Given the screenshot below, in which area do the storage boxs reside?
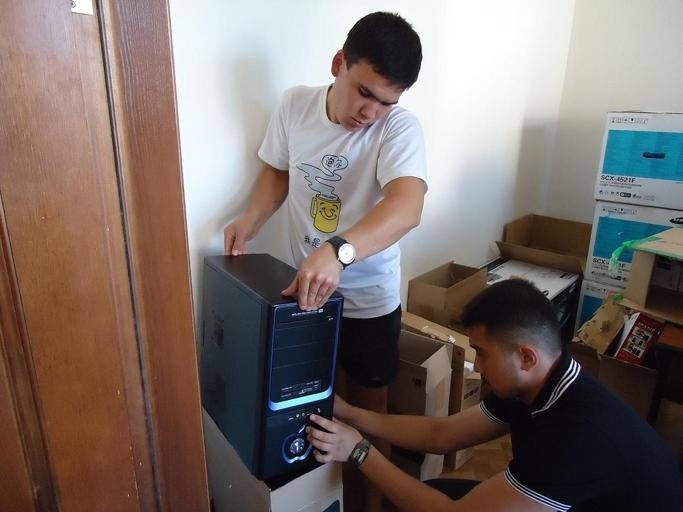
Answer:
[593,108,683,210]
[398,311,482,471]
[570,279,621,339]
[498,212,592,271]
[390,329,453,482]
[568,290,683,421]
[407,261,487,328]
[476,257,580,344]
[202,408,343,512]
[613,227,683,323]
[584,201,683,289]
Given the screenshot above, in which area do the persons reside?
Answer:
[222,10,427,512]
[304,276,683,511]
